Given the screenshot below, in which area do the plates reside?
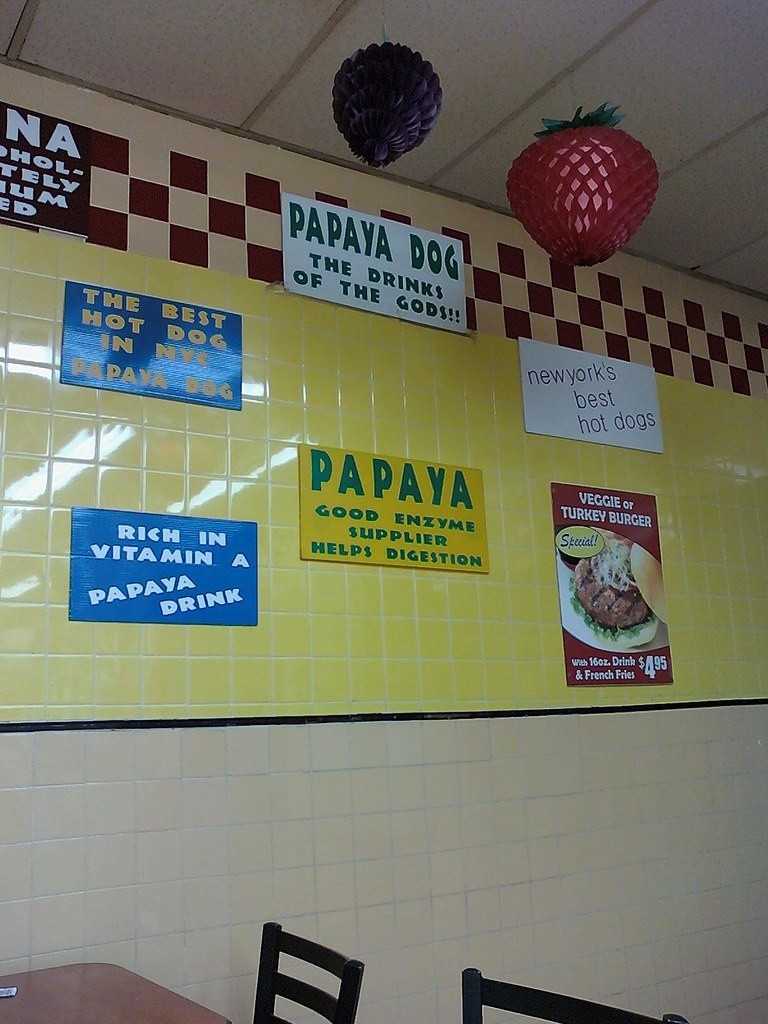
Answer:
[556,527,670,653]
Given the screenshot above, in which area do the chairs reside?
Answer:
[462,967,687,1024]
[249,922,366,1024]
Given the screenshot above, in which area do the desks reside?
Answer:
[0,962,232,1024]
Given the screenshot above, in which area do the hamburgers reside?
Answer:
[569,540,668,652]
